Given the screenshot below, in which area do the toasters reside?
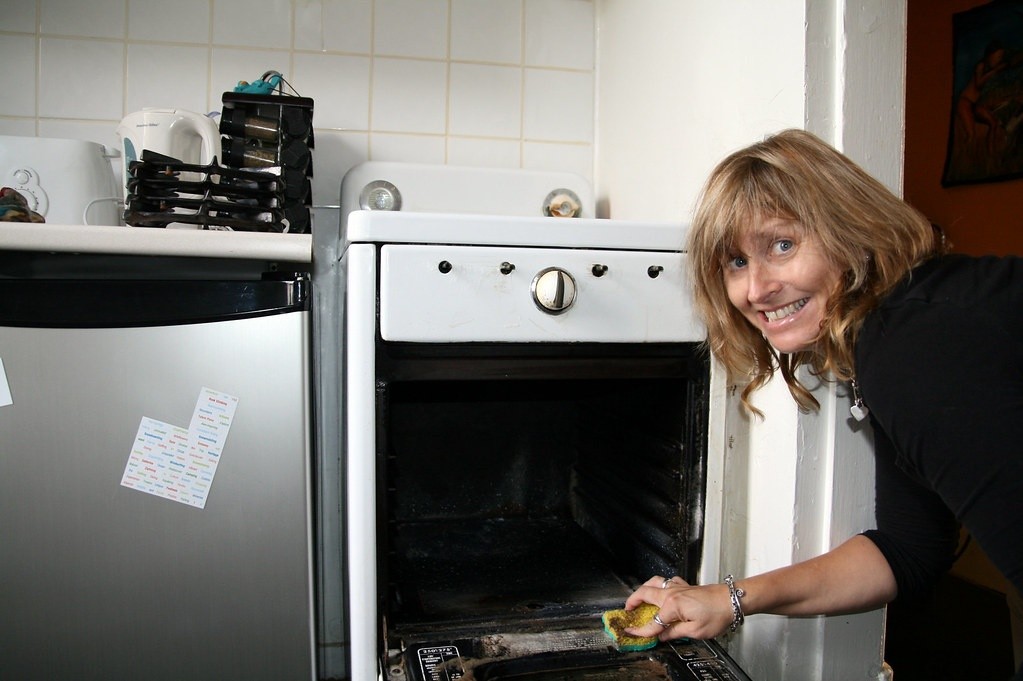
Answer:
[0,135,120,225]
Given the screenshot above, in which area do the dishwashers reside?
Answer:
[0,273,326,681]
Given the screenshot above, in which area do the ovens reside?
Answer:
[345,209,746,680]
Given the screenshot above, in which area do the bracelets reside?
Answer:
[724,575,745,633]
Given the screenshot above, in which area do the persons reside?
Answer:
[625,131,1023,681]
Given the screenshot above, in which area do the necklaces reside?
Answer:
[850,370,868,422]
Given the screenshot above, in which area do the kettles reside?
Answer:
[116,108,221,230]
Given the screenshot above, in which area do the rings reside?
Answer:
[653,615,670,629]
[662,578,672,588]
